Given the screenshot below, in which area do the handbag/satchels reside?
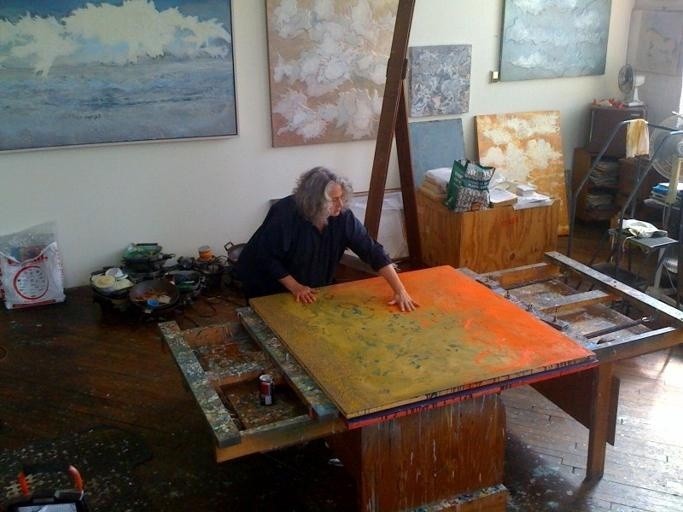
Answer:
[0,241,67,310]
[611,218,668,266]
[446,159,495,213]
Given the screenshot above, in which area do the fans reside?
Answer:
[617,64,646,107]
[644,114,683,291]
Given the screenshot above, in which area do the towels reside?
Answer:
[424,166,452,191]
[624,118,649,160]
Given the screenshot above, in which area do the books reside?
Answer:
[584,160,619,212]
[418,168,451,202]
[489,185,536,208]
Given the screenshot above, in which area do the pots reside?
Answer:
[88,240,248,323]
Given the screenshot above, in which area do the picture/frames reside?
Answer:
[0,2,241,157]
[266,186,412,265]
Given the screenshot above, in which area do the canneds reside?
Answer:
[259,373,276,407]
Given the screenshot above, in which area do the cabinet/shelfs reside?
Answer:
[571,146,619,223]
[586,103,647,154]
[616,158,667,222]
[412,189,561,274]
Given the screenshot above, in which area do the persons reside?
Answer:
[237,166,421,312]
